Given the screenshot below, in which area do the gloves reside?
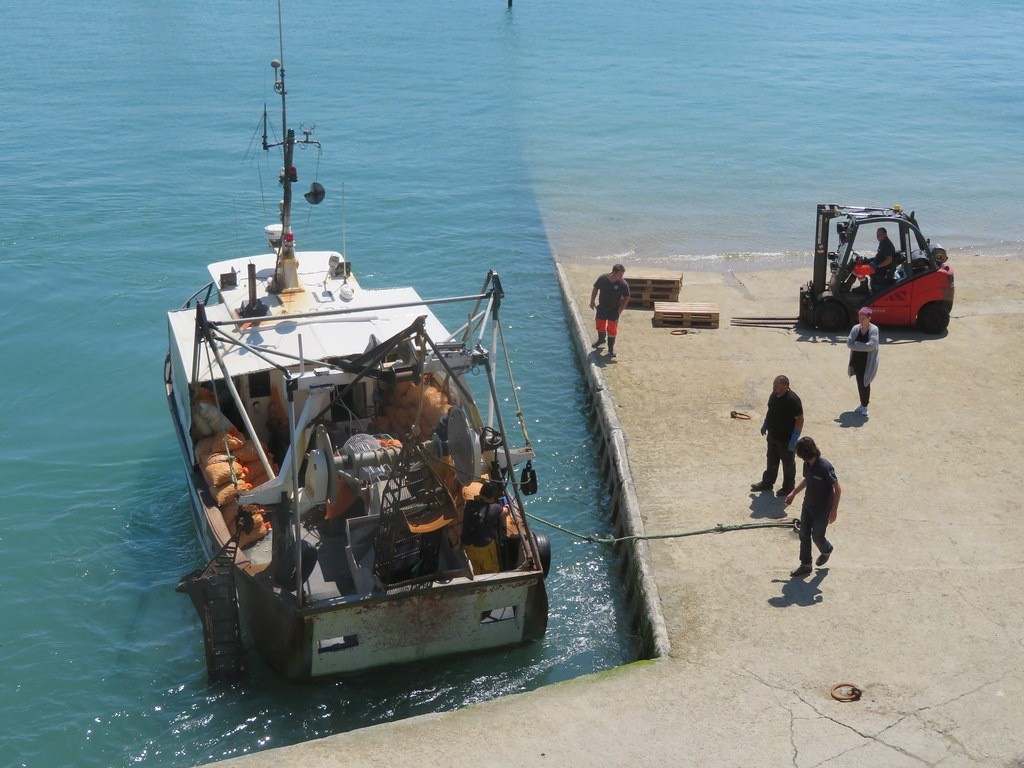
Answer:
[761,416,768,435]
[869,262,878,270]
[788,429,800,452]
[863,256,872,263]
[503,496,508,505]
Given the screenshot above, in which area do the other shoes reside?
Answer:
[776,488,795,496]
[751,481,773,490]
[790,565,812,576]
[862,407,868,415]
[855,405,862,411]
[816,546,833,566]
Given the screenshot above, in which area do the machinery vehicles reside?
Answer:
[731,202,954,336]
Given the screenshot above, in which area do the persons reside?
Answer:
[460,482,511,575]
[845,307,879,415]
[751,376,804,495]
[785,436,841,576]
[589,264,630,357]
[852,228,895,293]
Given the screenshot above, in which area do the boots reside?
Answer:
[592,331,606,347]
[608,337,616,356]
[852,277,869,294]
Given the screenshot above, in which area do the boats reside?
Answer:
[165,1,552,682]
